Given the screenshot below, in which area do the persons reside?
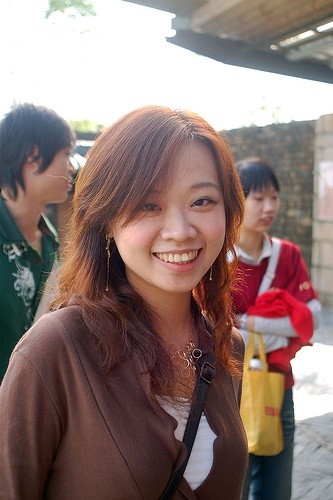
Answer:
[1,102,77,379]
[0,105,249,500]
[224,158,323,500]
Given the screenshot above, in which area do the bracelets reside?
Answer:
[240,313,247,329]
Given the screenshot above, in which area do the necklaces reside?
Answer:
[156,314,202,379]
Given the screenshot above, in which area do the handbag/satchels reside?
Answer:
[237,331,285,456]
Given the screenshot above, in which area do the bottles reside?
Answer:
[249,355,264,371]
